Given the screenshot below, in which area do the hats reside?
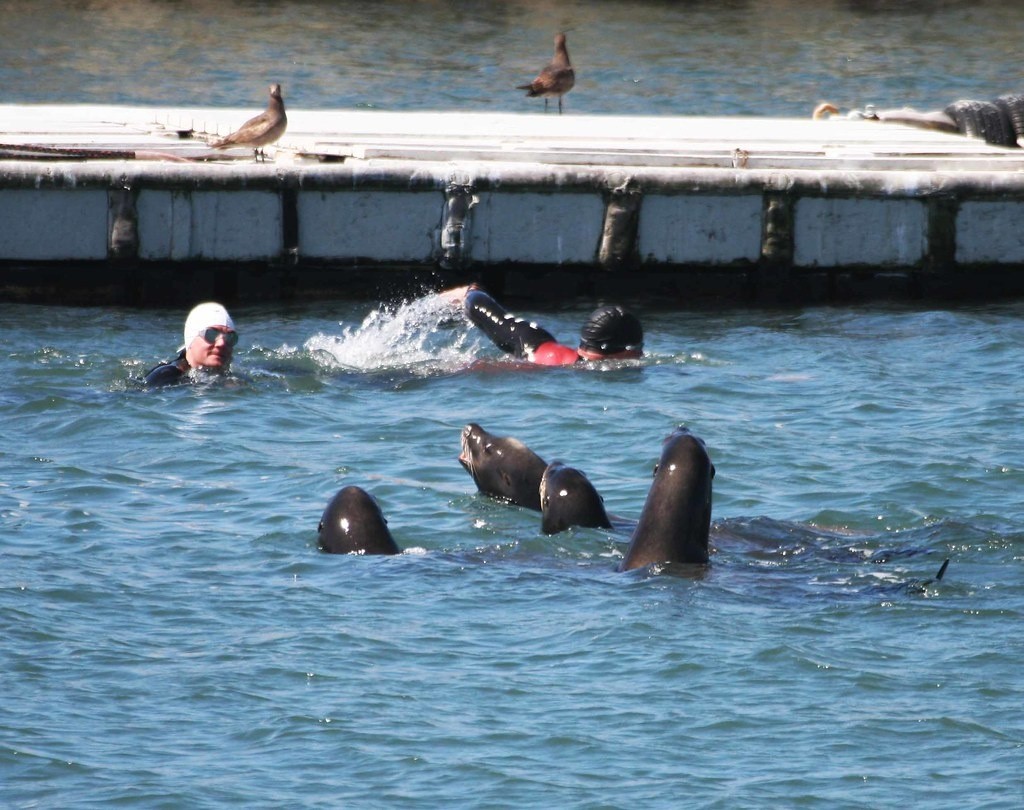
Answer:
[583,305,641,355]
[180,301,234,349]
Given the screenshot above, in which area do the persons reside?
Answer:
[419,285,644,372]
[125,301,241,392]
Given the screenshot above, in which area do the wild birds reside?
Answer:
[208,82,289,163]
[513,34,574,114]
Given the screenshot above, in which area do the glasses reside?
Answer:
[198,325,239,347]
[628,342,643,356]
